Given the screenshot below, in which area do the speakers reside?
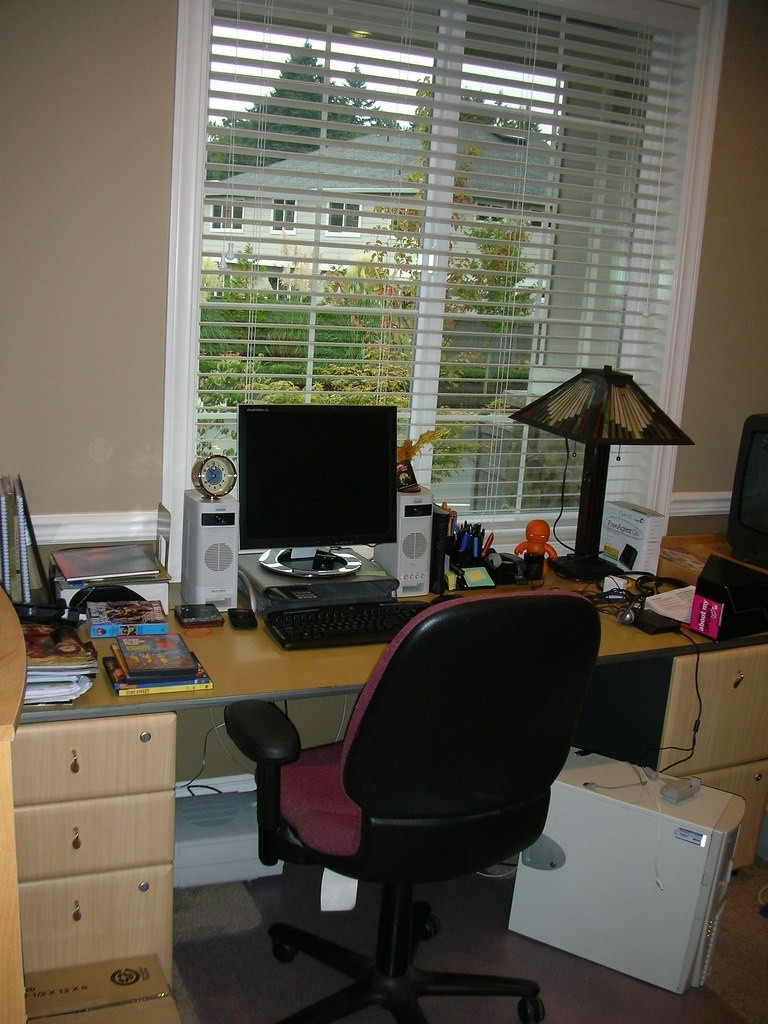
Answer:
[374,485,434,597]
[181,489,237,613]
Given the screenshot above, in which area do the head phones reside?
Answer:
[589,588,646,624]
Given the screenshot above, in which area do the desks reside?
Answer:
[12,572,768,985]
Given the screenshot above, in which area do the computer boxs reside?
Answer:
[508,745,746,995]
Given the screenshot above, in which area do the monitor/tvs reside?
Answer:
[236,404,398,578]
[727,413,768,569]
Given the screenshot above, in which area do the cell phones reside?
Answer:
[228,608,258,629]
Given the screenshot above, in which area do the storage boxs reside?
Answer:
[23,952,180,1024]
[599,500,669,579]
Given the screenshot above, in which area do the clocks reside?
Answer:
[191,455,237,499]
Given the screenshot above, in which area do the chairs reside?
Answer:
[221,591,602,1024]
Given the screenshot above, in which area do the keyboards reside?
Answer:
[263,601,430,650]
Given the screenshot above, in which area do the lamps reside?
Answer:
[512,363,694,583]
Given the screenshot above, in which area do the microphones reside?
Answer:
[621,597,637,620]
